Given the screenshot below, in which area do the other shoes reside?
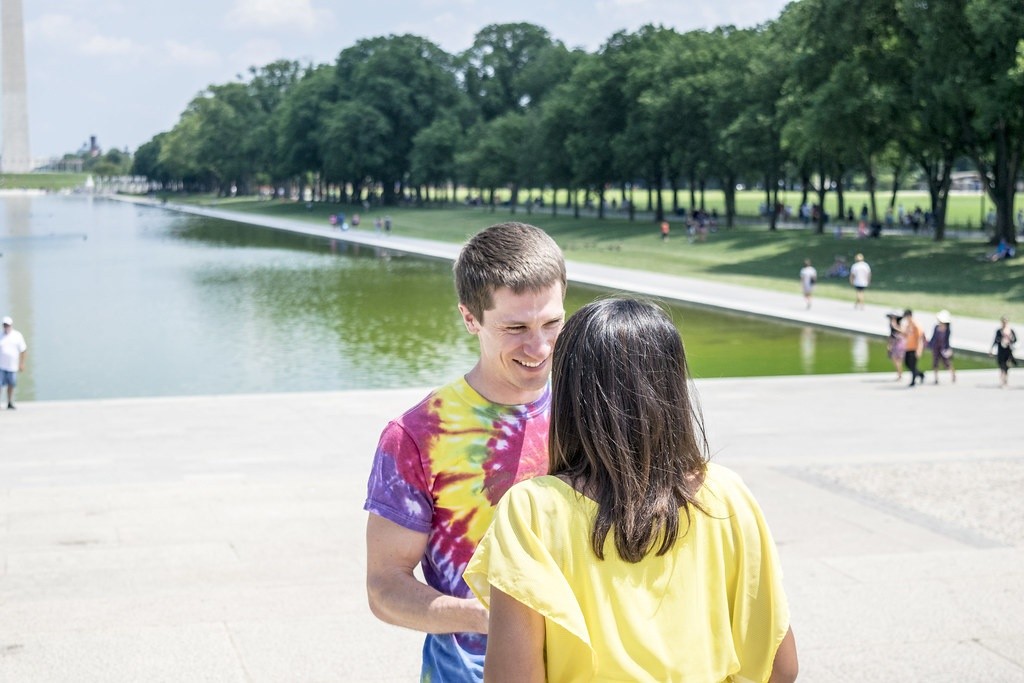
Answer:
[8,403,16,409]
[920,374,924,382]
[952,375,955,381]
[908,383,915,386]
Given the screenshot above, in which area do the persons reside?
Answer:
[363,222,566,683]
[849,253,871,308]
[887,310,956,386]
[758,200,820,223]
[0,316,27,408]
[986,238,1016,261]
[462,298,799,683]
[848,203,869,237]
[327,211,392,236]
[661,217,708,244]
[883,205,932,226]
[800,258,817,309]
[992,316,1017,386]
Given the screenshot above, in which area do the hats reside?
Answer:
[2,316,13,326]
[886,310,903,319]
[936,310,951,323]
[903,309,912,317]
